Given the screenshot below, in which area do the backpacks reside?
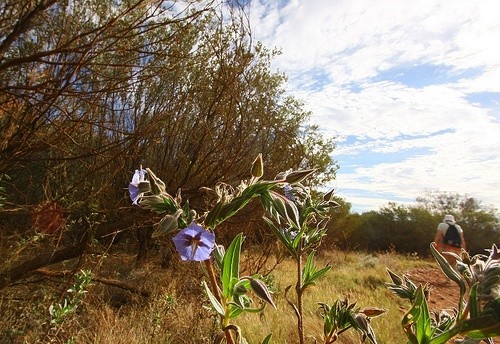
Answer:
[442,222,462,248]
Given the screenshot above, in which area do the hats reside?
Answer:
[441,214,456,223]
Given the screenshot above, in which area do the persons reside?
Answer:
[434,214,466,284]
